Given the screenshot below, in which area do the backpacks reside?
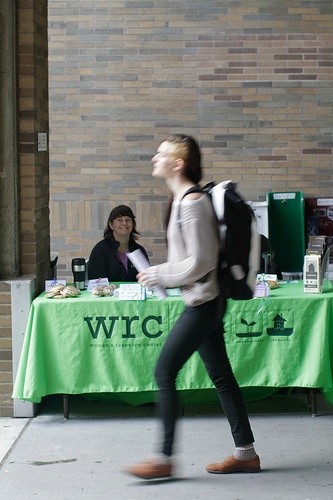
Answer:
[177,179,260,301]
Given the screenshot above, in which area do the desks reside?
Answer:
[11,280,333,418]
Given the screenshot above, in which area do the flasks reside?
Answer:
[72,258,86,290]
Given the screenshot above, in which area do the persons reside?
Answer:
[86,204,149,282]
[126,134,261,479]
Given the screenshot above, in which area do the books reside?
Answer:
[303,236,327,293]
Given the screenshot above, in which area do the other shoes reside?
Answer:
[205,455,261,474]
[122,459,171,479]
